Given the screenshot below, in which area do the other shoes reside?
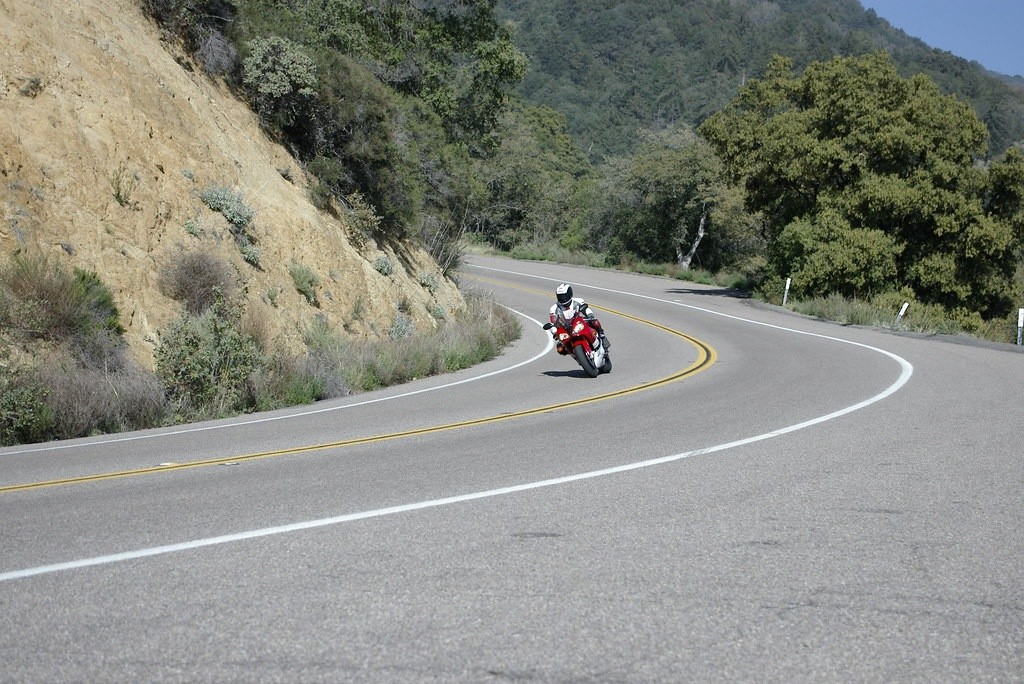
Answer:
[601,336,609,349]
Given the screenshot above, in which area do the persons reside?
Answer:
[548,283,605,366]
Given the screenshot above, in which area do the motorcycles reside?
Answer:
[543,303,612,377]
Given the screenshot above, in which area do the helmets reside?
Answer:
[555,284,573,307]
